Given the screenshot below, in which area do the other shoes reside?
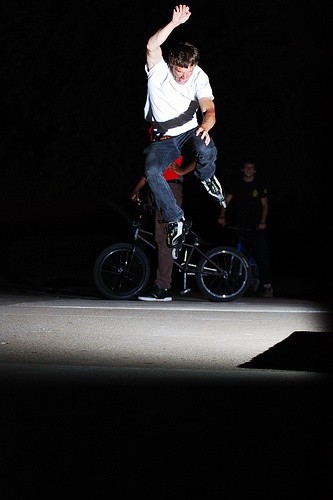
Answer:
[263,283,273,298]
[138,291,173,301]
[165,221,193,259]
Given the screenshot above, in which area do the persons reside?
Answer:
[140,5,226,247]
[218,160,274,299]
[130,123,195,302]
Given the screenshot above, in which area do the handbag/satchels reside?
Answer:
[145,100,198,141]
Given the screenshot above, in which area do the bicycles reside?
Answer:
[93,195,252,302]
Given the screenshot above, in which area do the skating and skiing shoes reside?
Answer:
[201,175,226,209]
